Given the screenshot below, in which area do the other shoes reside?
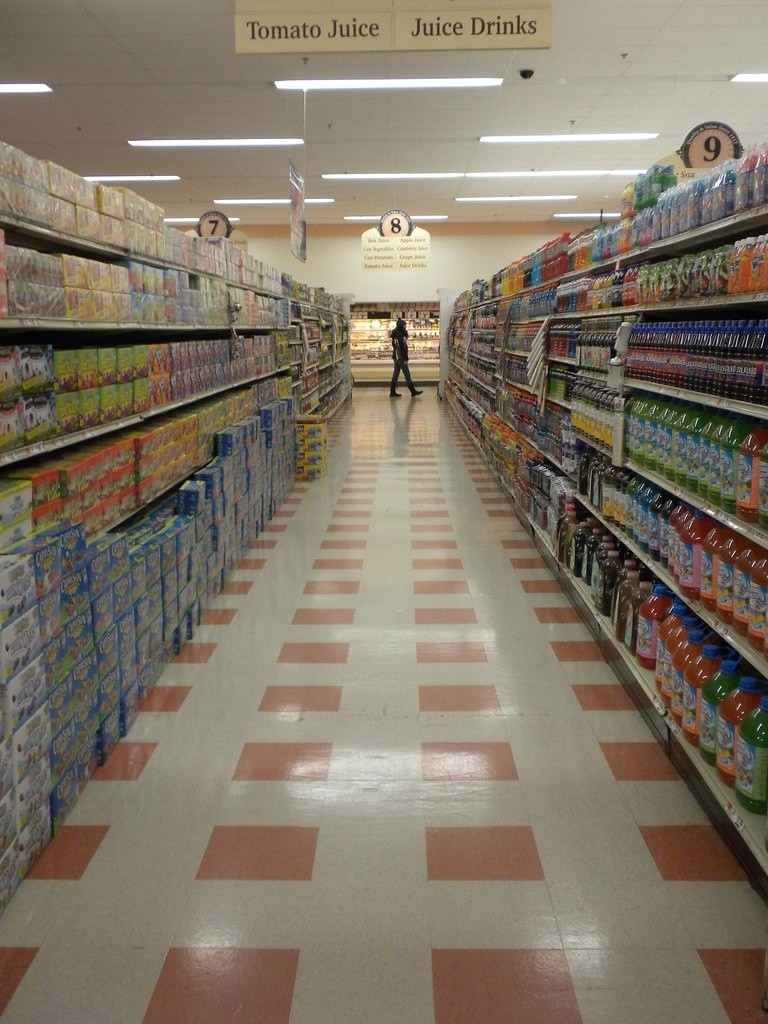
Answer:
[390,393,401,397]
[411,391,423,397]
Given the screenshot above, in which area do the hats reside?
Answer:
[397,318,406,328]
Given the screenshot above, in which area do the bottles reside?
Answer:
[443,138,768,818]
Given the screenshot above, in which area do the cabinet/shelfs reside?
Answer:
[350,204,768,907]
[0,212,353,543]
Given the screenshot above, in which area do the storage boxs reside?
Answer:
[0,141,331,913]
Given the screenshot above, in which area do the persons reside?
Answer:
[389,317,424,397]
